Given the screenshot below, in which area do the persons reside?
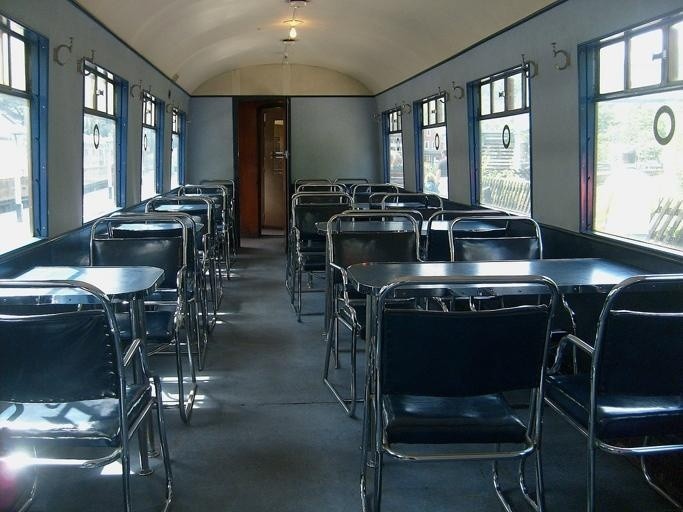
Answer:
[594,149,660,239]
[435,150,448,199]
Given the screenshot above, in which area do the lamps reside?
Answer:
[282,6,304,38]
